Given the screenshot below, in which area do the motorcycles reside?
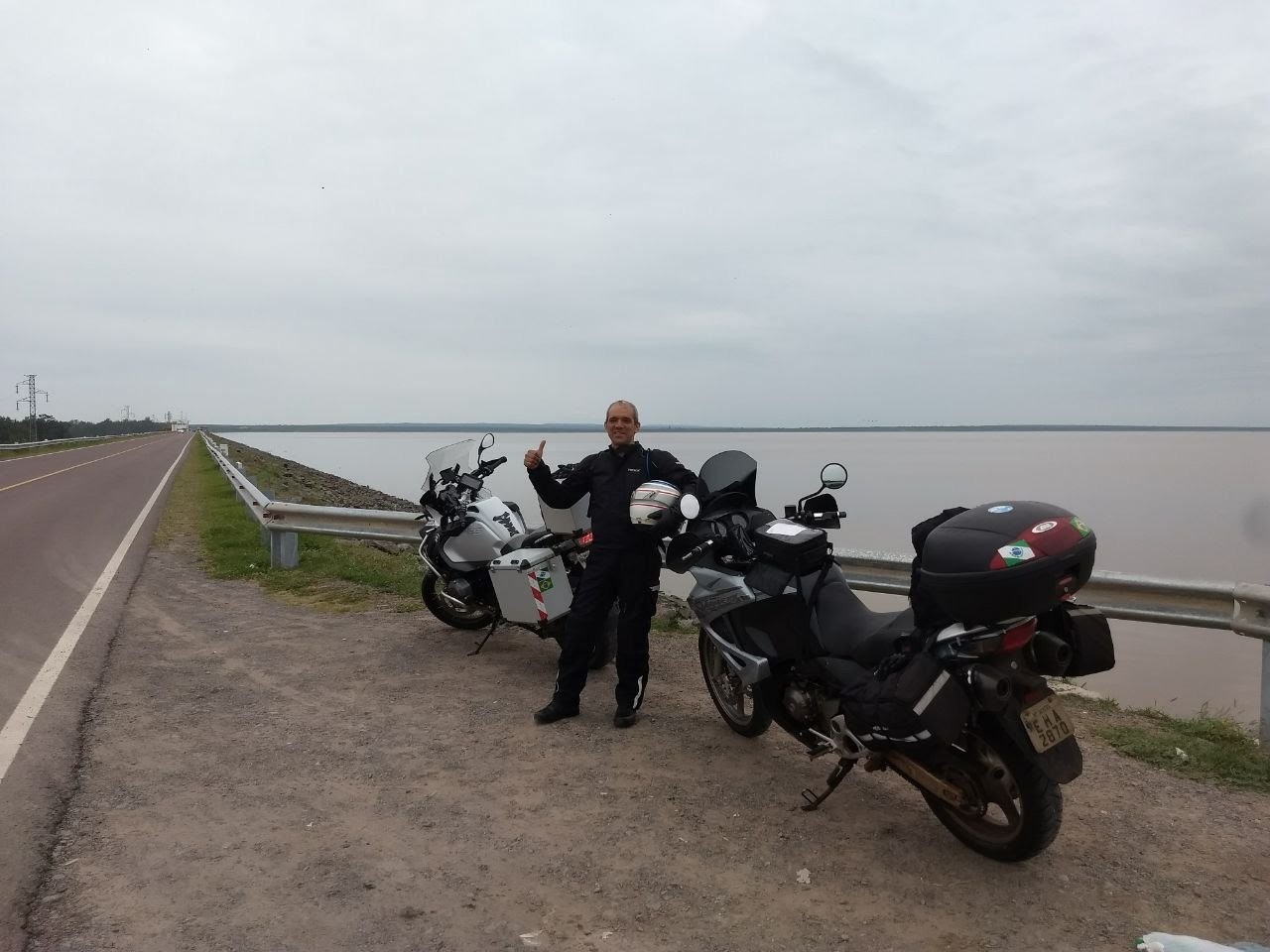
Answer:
[663,449,1097,863]
[413,433,620,670]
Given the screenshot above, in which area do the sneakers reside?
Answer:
[614,709,637,728]
[534,703,580,723]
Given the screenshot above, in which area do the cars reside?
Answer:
[176,428,192,434]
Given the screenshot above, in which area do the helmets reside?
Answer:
[629,479,682,526]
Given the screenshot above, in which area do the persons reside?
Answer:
[524,399,711,729]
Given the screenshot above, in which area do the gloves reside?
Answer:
[649,498,684,540]
